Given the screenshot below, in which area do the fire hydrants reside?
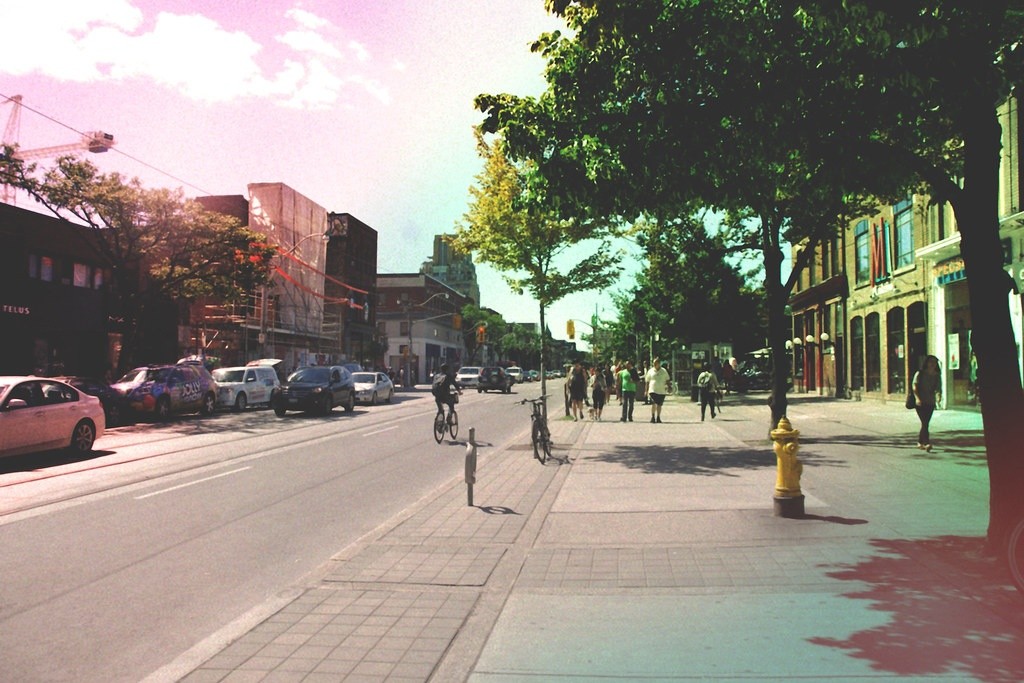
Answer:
[769,415,804,497]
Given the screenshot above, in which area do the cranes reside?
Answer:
[0,95,117,207]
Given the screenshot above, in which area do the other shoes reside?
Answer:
[620,416,627,421]
[579,414,584,419]
[650,417,656,423]
[574,416,577,422]
[656,417,661,423]
[712,413,716,418]
[923,443,932,452]
[447,421,454,425]
[917,442,923,448]
[628,415,633,421]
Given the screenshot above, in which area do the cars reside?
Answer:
[350,372,394,405]
[454,367,483,389]
[270,366,356,417]
[0,374,106,457]
[523,370,565,382]
[506,366,523,384]
[125,355,222,422]
[43,376,124,429]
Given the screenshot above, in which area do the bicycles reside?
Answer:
[514,394,554,464]
[433,393,463,444]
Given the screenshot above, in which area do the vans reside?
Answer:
[211,358,284,413]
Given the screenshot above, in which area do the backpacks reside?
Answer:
[698,371,712,391]
[431,374,450,398]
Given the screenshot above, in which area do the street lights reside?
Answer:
[410,293,449,386]
[258,232,329,359]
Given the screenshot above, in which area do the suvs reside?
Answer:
[476,367,511,394]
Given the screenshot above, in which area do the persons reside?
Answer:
[697,363,720,421]
[619,361,639,422]
[362,362,415,392]
[723,354,739,395]
[590,368,607,421]
[912,355,942,452]
[645,358,672,423]
[435,363,463,425]
[595,360,650,406]
[566,359,590,421]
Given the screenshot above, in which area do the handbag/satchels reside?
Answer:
[905,391,915,409]
